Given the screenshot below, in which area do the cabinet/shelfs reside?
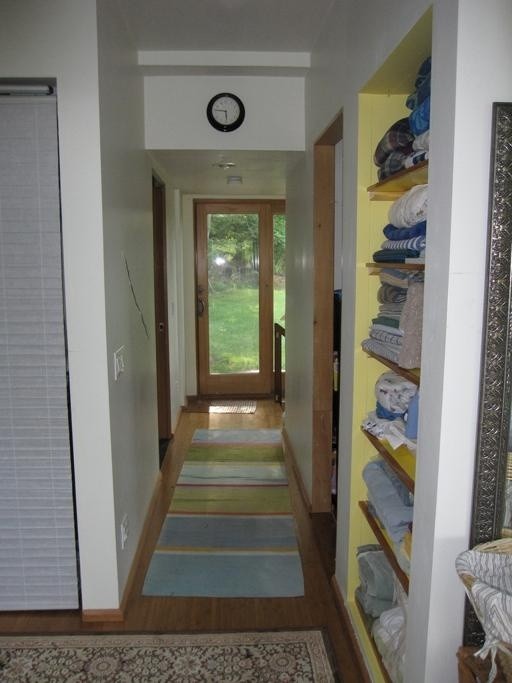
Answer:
[355,160,427,682]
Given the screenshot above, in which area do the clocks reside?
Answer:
[207,93,245,132]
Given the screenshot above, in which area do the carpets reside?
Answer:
[1,624,345,682]
[140,429,306,598]
[183,399,257,413]
